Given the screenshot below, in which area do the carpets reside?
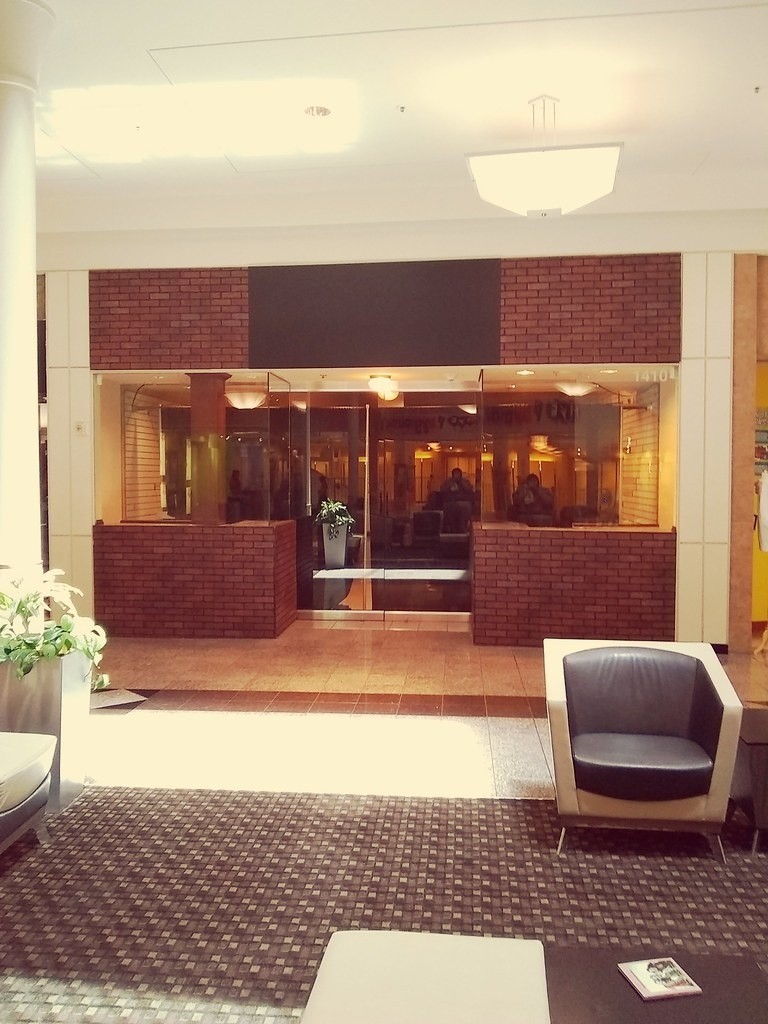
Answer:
[0,786,768,1024]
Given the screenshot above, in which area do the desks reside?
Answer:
[299,929,768,1024]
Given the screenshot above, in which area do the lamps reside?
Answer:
[555,383,599,397]
[368,375,393,391]
[224,382,269,409]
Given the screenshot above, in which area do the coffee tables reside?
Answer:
[725,708,768,856]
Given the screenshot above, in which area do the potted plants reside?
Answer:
[315,498,356,567]
[0,560,111,816]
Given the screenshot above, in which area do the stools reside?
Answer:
[439,533,467,559]
[0,731,57,853]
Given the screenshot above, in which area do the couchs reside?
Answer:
[412,511,443,540]
[543,638,742,864]
[359,512,411,552]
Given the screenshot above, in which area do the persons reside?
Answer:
[512,474,554,528]
[291,472,304,519]
[230,469,243,496]
[317,476,328,506]
[440,466,476,533]
